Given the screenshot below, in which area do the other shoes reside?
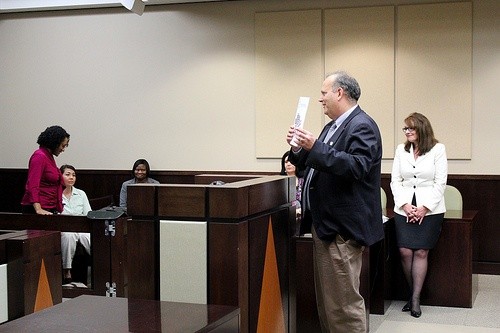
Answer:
[65,277,71,283]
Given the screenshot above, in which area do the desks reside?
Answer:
[0,293,241,333]
[390,208,478,309]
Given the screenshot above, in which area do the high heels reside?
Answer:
[402,300,421,318]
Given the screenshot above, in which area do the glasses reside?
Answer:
[402,125,416,132]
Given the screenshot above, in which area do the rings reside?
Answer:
[418,218,420,220]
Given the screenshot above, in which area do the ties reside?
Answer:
[302,122,336,218]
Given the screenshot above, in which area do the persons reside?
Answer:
[57,165,92,284]
[120,159,160,213]
[280,150,304,216]
[390,112,448,318]
[19,126,70,215]
[286,71,385,333]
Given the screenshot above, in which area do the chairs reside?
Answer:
[380,187,387,209]
[444,185,463,211]
